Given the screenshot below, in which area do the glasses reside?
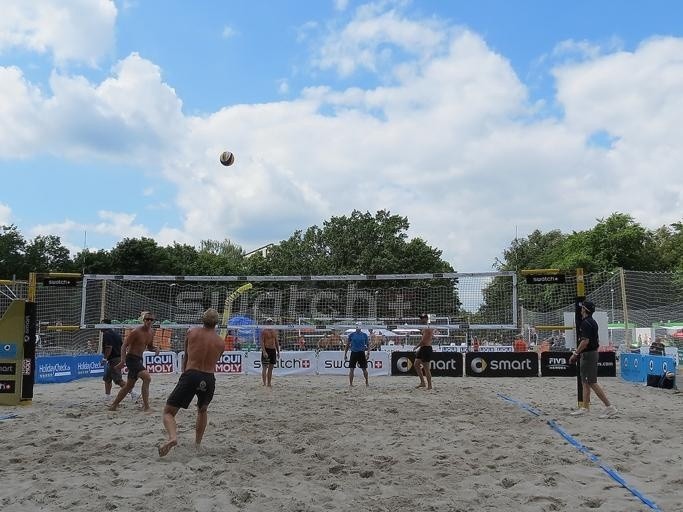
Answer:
[144,318,154,321]
[267,320,272,322]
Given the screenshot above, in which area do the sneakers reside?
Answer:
[598,405,620,419]
[569,407,591,416]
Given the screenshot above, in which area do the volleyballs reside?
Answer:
[219,151,235,166]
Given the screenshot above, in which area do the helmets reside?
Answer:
[355,321,362,329]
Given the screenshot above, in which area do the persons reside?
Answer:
[513,334,528,352]
[259,317,281,387]
[344,322,370,387]
[569,301,621,419]
[100,311,159,412]
[158,308,226,457]
[649,335,666,356]
[224,329,242,351]
[297,327,378,351]
[412,314,434,390]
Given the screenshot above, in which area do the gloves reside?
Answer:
[100,358,107,367]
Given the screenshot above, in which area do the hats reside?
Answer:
[418,315,429,318]
[578,300,595,314]
